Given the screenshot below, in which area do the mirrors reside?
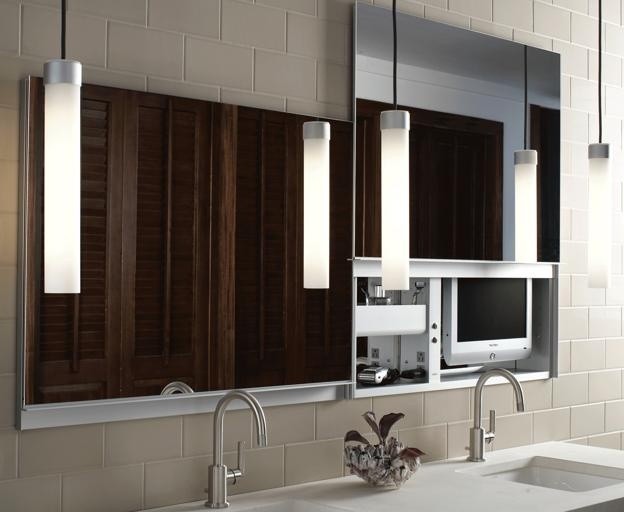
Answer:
[24,77,353,406]
[352,1,561,263]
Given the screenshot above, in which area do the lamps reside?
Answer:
[514,46,537,262]
[379,0,411,291]
[43,0,83,295]
[303,121,331,290]
[588,0,609,289]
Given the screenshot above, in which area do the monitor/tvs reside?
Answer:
[442,278,533,366]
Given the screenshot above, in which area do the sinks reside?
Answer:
[454,455,624,492]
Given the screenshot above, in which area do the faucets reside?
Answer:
[160,381,194,396]
[474,368,524,428]
[213,390,268,466]
